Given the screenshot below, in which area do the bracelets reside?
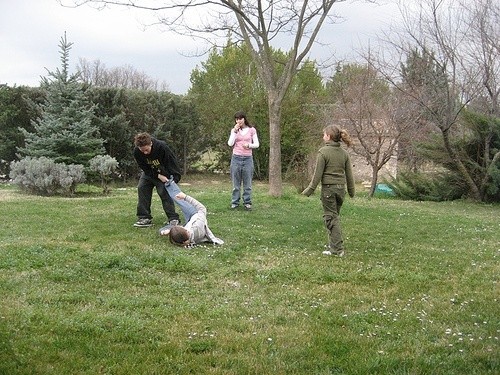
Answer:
[184,196,188,201]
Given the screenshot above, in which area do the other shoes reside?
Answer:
[231,203,239,208]
[244,203,251,208]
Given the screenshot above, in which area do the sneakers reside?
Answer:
[133,218,152,226]
[167,220,181,225]
[322,250,345,258]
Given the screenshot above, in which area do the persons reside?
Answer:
[152,165,224,248]
[227,111,259,210]
[133,132,182,226]
[301,125,355,257]
